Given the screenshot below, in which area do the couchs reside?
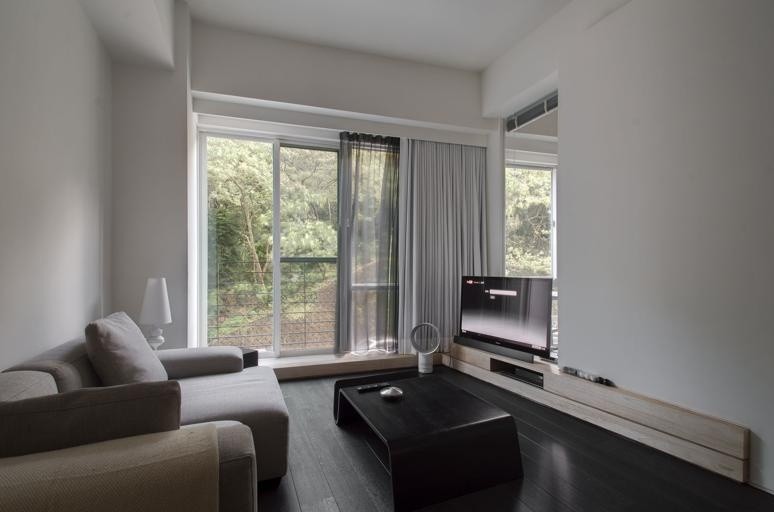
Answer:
[0,334,289,512]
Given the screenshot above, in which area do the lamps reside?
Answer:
[138,276,172,347]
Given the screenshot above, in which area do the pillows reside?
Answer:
[86,312,167,388]
[0,382,183,460]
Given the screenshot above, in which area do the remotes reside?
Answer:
[357,381,391,393]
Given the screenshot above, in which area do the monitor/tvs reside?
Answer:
[461,274,553,359]
